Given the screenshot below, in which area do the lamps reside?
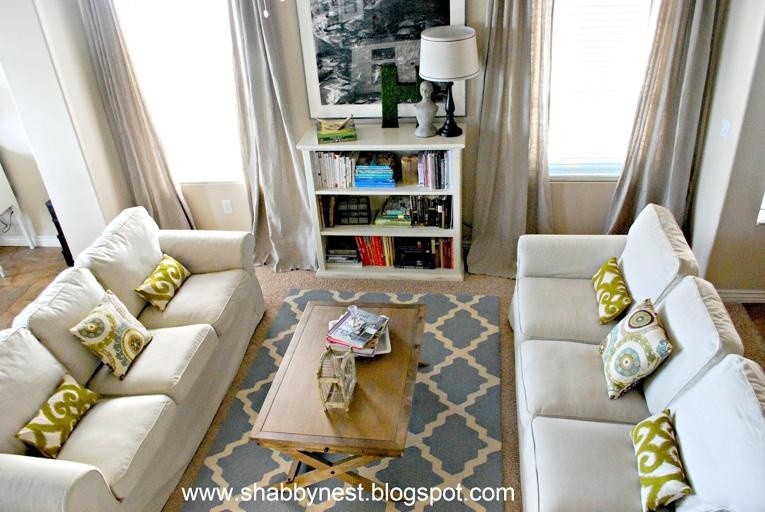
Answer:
[416,22,484,137]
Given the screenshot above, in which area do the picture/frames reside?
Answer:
[295,0,469,119]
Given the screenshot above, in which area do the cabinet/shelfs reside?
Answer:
[295,123,468,283]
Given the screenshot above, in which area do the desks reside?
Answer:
[249,300,427,502]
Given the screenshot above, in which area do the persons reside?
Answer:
[413,80,439,129]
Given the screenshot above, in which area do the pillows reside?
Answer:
[596,294,674,403]
[133,254,194,315]
[591,255,632,325]
[14,370,102,461]
[629,404,696,512]
[68,287,152,380]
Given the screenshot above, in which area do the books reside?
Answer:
[326,307,390,357]
[309,152,455,269]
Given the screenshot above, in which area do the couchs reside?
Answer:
[505,201,765,512]
[0,203,268,512]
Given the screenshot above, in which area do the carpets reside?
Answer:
[179,285,505,512]
